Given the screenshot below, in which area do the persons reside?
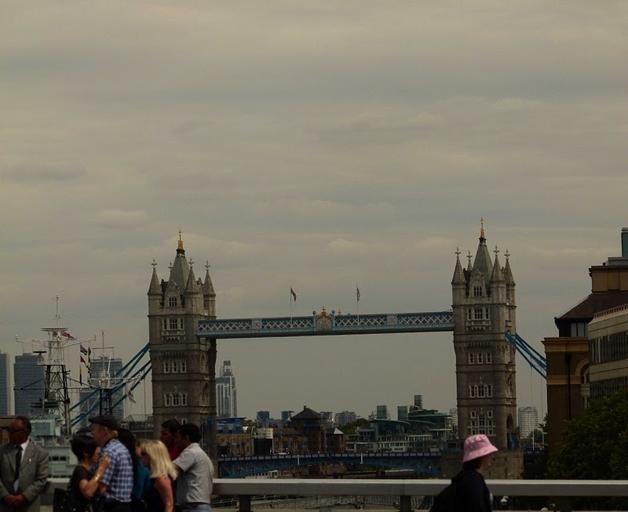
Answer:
[432,433,498,512]
[0,415,51,512]
[66,413,214,512]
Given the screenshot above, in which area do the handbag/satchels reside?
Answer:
[52,488,106,512]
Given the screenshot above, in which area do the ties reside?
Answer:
[12,446,22,496]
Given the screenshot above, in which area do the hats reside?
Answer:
[463,434,499,463]
[88,415,118,431]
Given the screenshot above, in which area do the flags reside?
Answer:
[290,286,296,303]
[355,287,361,304]
